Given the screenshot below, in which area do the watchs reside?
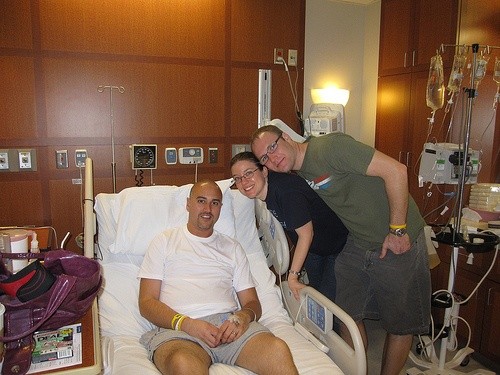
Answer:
[389,228,407,237]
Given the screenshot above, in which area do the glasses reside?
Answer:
[258,134,282,166]
[233,167,261,184]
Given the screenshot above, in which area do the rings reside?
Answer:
[232,331,237,335]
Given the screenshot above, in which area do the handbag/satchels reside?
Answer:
[0,248,102,375]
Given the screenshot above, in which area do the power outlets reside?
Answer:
[274,48,297,66]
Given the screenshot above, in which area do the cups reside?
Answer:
[0,229,29,274]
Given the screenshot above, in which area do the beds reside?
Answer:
[83,156,368,375]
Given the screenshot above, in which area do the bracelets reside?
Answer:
[389,223,407,230]
[171,314,189,331]
[289,270,300,276]
[243,308,256,322]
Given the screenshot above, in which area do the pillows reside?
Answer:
[108,176,239,253]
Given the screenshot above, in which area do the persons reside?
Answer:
[229,152,350,332]
[250,125,432,375]
[137,179,299,375]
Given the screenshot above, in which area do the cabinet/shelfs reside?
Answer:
[375,0,500,363]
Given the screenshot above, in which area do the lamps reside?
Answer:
[310,86,351,109]
